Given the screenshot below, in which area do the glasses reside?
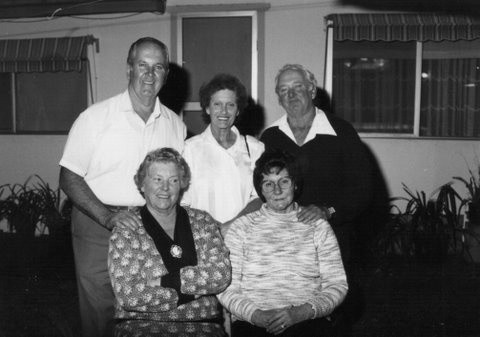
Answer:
[260,177,294,192]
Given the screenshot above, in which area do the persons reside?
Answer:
[108,146,231,336]
[57,38,188,337]
[216,150,351,337]
[174,72,267,224]
[263,62,387,337]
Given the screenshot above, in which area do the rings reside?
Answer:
[281,323,287,329]
